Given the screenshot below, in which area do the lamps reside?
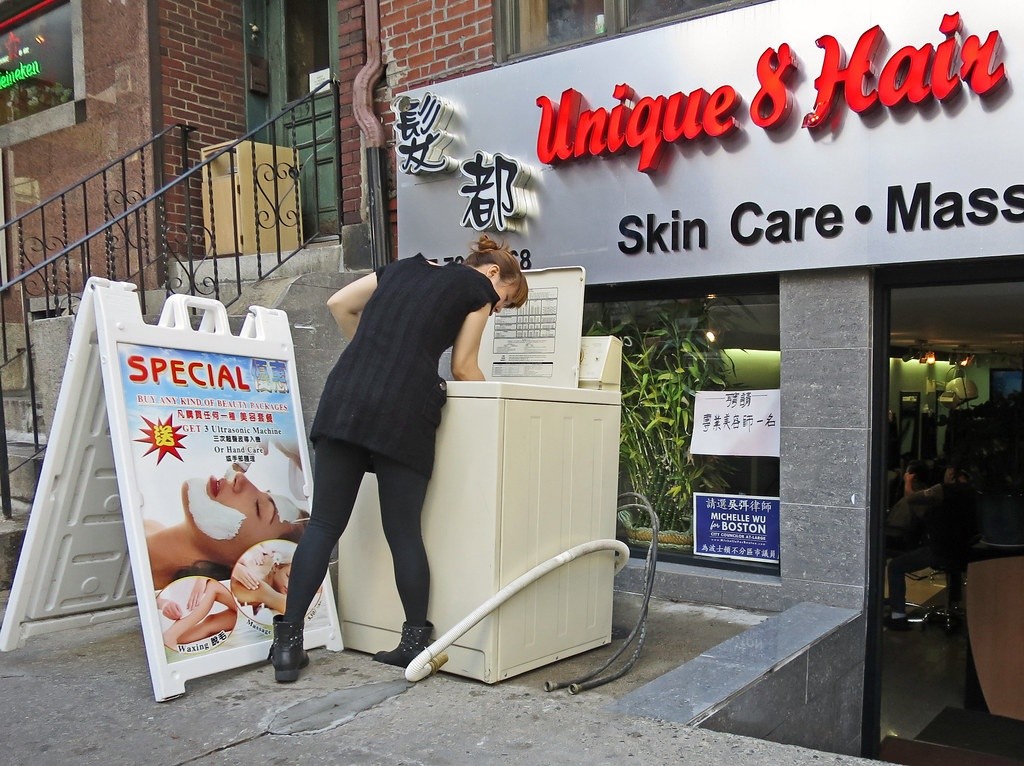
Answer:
[902,339,935,365]
[949,353,975,367]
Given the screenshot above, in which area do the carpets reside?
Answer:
[880,706,1024,766]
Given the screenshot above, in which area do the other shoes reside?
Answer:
[883,614,908,631]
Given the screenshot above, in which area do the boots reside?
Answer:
[268,614,310,683]
[372,619,433,669]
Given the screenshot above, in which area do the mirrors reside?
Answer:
[899,391,920,459]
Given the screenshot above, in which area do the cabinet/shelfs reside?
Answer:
[201,140,303,256]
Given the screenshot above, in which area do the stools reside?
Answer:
[907,555,966,635]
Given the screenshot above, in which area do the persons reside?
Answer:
[269,235,528,684]
[144,430,322,650]
[883,458,984,634]
[886,459,929,560]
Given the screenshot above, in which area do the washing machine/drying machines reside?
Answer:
[329,264,627,686]
